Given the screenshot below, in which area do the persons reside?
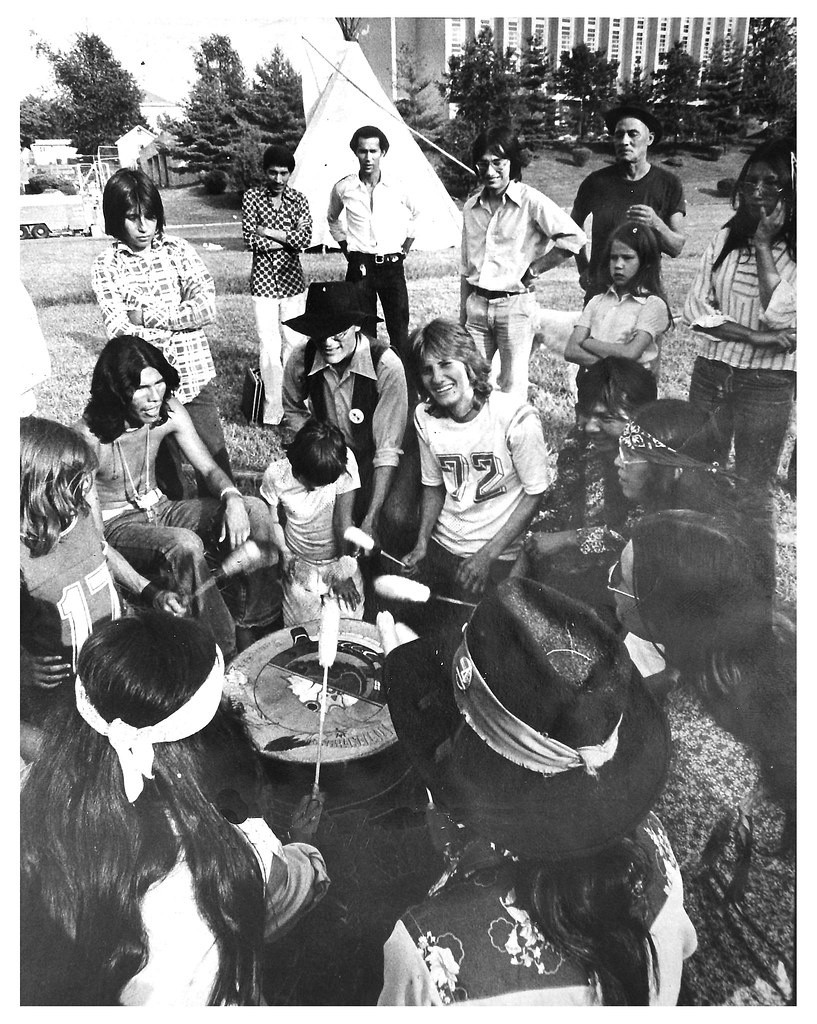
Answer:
[683,133,797,482]
[328,126,421,355]
[374,576,700,1005]
[571,104,687,307]
[242,145,312,426]
[20,282,797,1007]
[460,129,588,403]
[90,167,234,499]
[20,615,330,1007]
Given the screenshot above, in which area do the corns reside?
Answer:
[219,526,430,668]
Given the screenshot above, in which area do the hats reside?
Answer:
[604,102,663,144]
[280,280,383,339]
[382,574,673,854]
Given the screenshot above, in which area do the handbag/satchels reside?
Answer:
[242,368,264,427]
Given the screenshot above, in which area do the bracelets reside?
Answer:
[220,487,242,500]
[526,262,538,278]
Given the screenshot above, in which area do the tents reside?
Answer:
[285,40,465,248]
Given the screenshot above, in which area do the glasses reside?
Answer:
[606,560,640,601]
[331,328,347,340]
[474,159,511,173]
[735,180,783,202]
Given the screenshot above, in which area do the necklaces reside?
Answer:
[460,407,474,420]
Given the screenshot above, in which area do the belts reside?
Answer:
[472,286,521,298]
[347,251,403,264]
